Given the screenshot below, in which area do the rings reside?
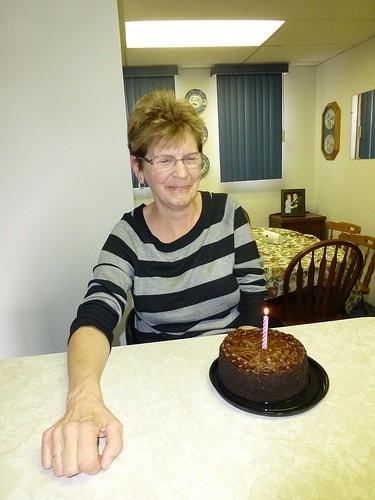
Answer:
[52,452,62,457]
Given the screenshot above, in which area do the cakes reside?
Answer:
[218,329,310,402]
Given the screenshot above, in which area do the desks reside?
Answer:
[267,212,326,241]
[253,228,354,300]
[0,316,375,500]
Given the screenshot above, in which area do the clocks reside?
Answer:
[320,102,341,160]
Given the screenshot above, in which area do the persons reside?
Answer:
[41,89,267,477]
[285,193,300,213]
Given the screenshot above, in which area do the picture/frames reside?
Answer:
[280,188,306,217]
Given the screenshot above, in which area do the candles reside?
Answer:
[261,308,269,349]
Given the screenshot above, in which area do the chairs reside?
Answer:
[282,221,375,326]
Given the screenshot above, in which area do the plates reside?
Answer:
[201,124,208,144]
[201,154,210,175]
[209,356,329,417]
[185,88,207,112]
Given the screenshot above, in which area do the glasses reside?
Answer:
[139,152,203,169]
